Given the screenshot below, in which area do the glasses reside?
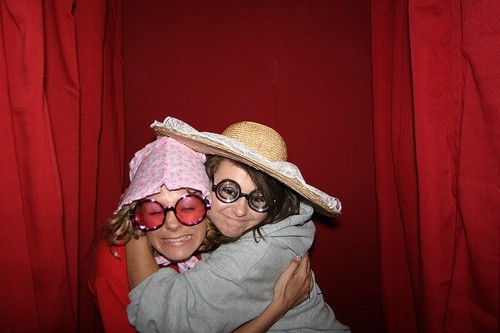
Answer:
[130,194,210,232]
[211,173,276,213]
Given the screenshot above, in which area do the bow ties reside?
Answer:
[153,250,199,272]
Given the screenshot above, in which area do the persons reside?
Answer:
[121,116,354,333]
[83,136,315,333]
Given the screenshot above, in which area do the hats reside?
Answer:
[114,137,211,215]
[153,115,341,217]
